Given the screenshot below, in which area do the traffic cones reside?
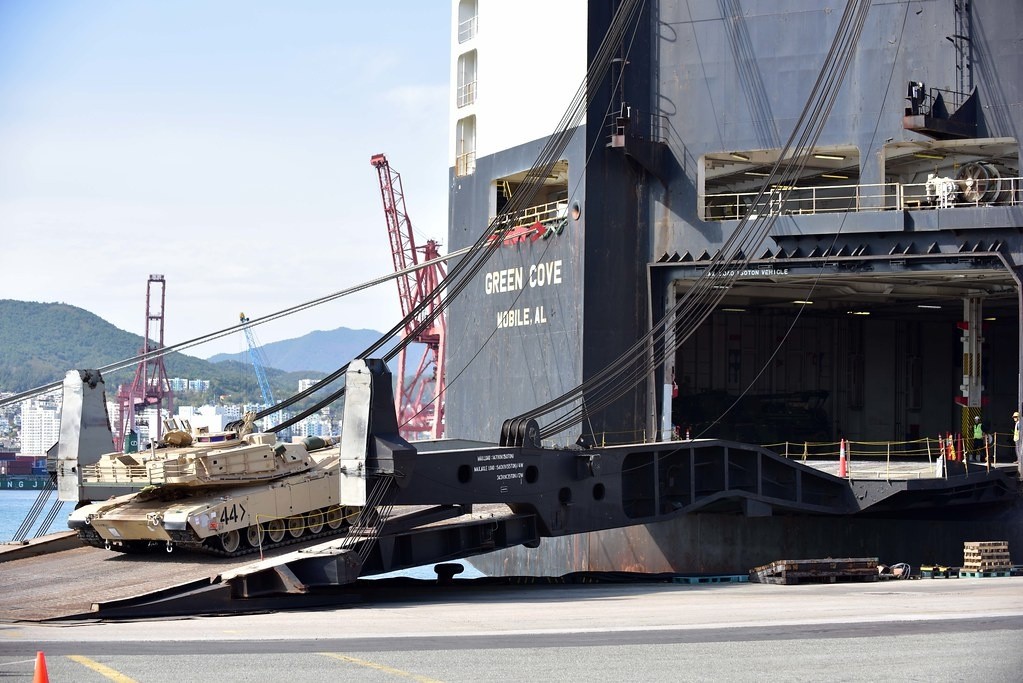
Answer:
[839,438,847,478]
[32,650,50,683]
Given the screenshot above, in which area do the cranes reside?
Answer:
[239,314,286,445]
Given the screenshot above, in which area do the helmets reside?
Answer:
[973,416,980,419]
[1012,412,1020,418]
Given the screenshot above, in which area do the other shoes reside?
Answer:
[1013,460,1019,464]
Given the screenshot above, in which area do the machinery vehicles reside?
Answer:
[67,430,364,557]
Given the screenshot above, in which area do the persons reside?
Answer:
[1013,412,1023,464]
[970,416,988,463]
